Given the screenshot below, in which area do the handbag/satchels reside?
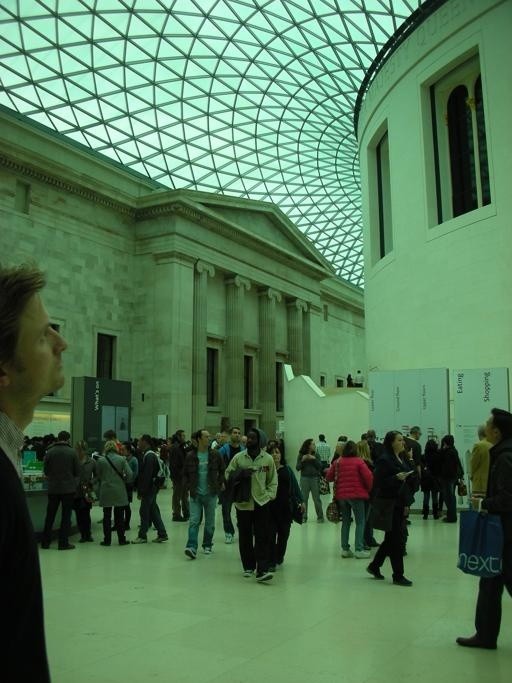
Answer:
[327,502,341,524]
[318,475,330,495]
[294,497,306,524]
[455,498,504,578]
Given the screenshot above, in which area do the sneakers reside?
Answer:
[393,576,412,587]
[420,508,459,523]
[367,564,384,580]
[355,550,371,559]
[255,572,273,582]
[40,514,168,550]
[184,548,196,559]
[203,546,212,554]
[224,533,233,544]
[172,512,191,522]
[244,570,254,577]
[456,632,498,650]
[342,550,354,558]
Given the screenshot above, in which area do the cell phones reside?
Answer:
[405,470,414,474]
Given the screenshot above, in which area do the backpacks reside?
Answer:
[142,450,170,490]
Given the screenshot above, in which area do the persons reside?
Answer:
[0,262,67,683]
